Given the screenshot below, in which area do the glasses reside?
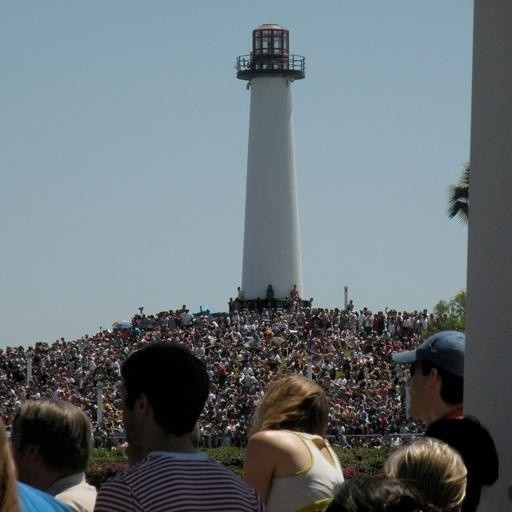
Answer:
[410,366,422,377]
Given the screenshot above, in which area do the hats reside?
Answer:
[392,331,466,378]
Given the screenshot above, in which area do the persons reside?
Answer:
[1,299,461,446]
[242,375,346,512]
[264,283,275,301]
[92,339,266,511]
[11,397,97,511]
[323,473,433,511]
[289,284,299,300]
[1,421,77,511]
[335,436,469,511]
[385,325,466,460]
[234,286,244,302]
